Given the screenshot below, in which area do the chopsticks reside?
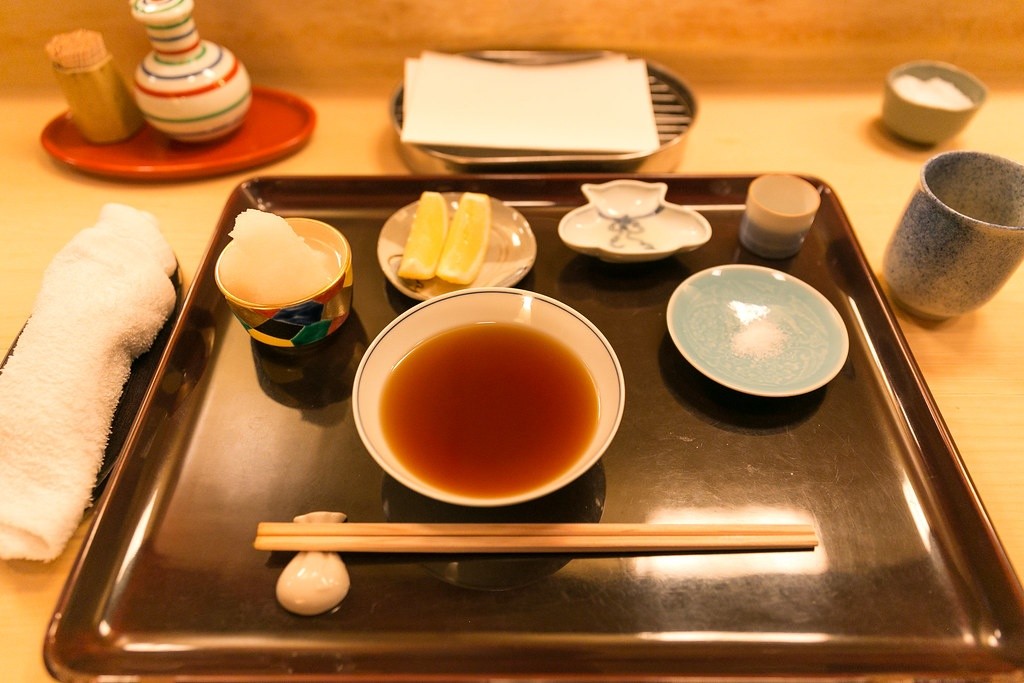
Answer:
[254,522,817,550]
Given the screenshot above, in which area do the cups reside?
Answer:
[883,150,1024,322]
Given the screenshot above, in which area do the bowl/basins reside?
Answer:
[882,61,987,144]
[215,219,354,350]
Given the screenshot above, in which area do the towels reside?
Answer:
[0,202,179,565]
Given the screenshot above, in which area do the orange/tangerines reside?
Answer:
[398,191,491,285]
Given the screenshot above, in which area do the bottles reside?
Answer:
[128,0,253,145]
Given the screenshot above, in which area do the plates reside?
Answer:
[559,179,712,262]
[377,191,537,301]
[666,264,849,397]
[0,255,184,492]
[352,288,625,509]
[42,86,315,181]
